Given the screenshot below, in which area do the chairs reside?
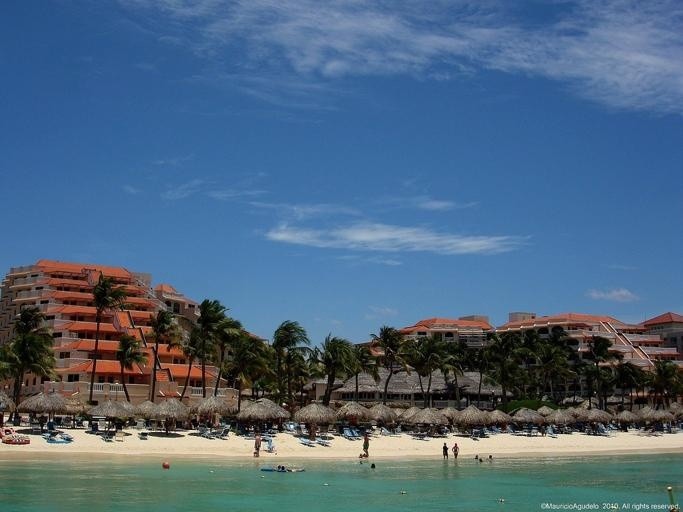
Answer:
[20,420,682,447]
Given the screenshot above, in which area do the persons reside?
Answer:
[363,433,369,455]
[452,443,459,459]
[475,455,478,459]
[443,443,449,460]
[276,465,282,472]
[489,455,492,459]
[255,432,261,457]
[479,458,483,462]
[281,466,287,472]
[268,435,273,451]
